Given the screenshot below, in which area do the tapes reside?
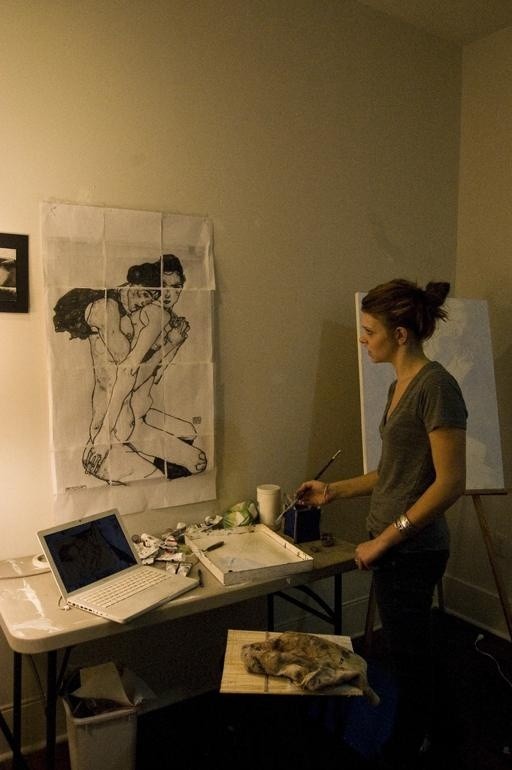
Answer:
[32,553,50,569]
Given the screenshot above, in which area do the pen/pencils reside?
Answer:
[175,562,181,575]
[207,541,224,552]
[198,569,204,588]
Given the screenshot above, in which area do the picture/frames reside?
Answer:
[0,232,30,311]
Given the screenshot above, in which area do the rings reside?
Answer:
[354,559,358,561]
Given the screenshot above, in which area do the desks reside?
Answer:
[0,522,377,770]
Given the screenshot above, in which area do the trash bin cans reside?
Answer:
[60,682,143,770]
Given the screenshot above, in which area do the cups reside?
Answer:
[256,484,282,532]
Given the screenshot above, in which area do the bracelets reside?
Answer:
[394,514,413,536]
[324,483,329,504]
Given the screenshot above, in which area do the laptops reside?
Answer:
[36,508,200,625]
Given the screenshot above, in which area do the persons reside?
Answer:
[295,279,468,768]
[86,253,208,475]
[53,262,191,484]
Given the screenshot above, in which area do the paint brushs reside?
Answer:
[275,449,344,525]
